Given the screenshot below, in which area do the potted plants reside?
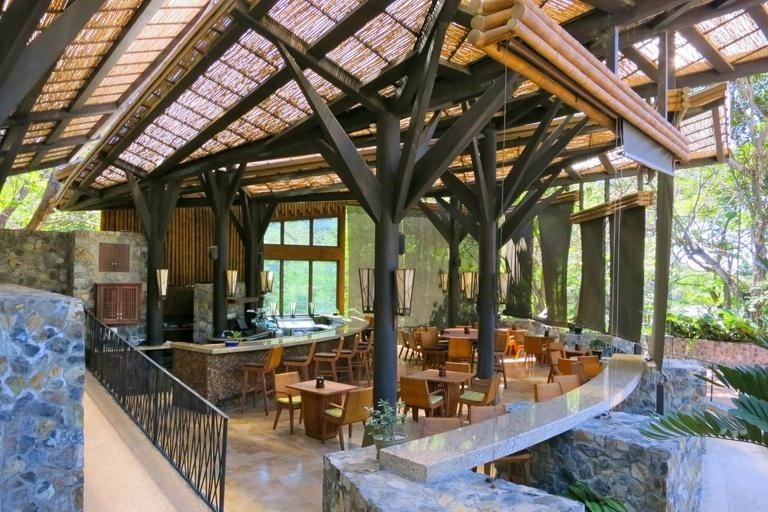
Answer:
[364,399,409,459]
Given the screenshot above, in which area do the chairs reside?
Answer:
[397,324,604,423]
[332,331,374,387]
[240,345,282,416]
[283,341,315,380]
[313,336,345,382]
[468,404,531,486]
[273,371,304,435]
[321,387,372,450]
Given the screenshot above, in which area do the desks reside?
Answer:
[285,377,360,440]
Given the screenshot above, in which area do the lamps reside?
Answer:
[260,270,273,293]
[442,268,511,304]
[395,269,416,316]
[156,268,168,300]
[226,270,238,303]
[359,268,375,312]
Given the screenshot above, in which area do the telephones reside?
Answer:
[128,335,140,347]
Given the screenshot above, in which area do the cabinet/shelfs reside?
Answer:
[99,242,130,272]
[94,282,142,325]
